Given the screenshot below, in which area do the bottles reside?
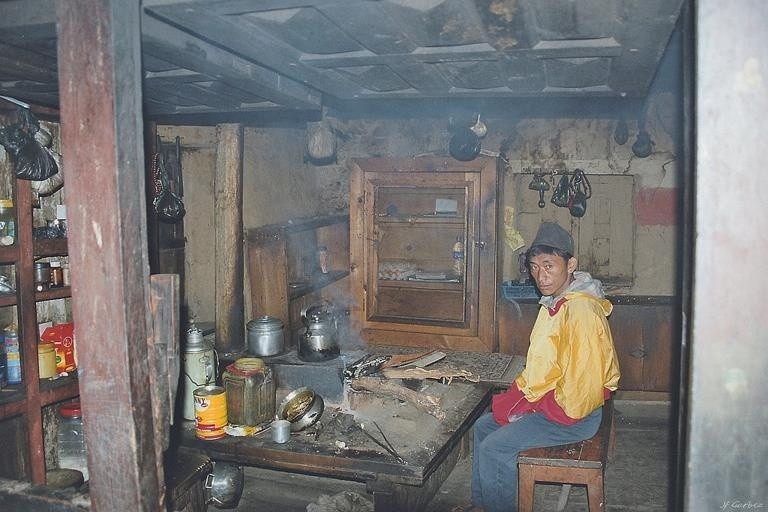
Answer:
[0,335,8,389]
[0,199,17,249]
[451,233,463,278]
[49,260,64,289]
[34,261,53,291]
[61,263,72,288]
[316,246,328,274]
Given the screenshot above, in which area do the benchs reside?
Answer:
[517,391,614,512]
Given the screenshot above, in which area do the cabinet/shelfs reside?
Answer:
[497,294,673,393]
[0,95,186,486]
[346,155,502,352]
[246,214,349,348]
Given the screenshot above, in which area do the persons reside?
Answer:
[463,221,626,510]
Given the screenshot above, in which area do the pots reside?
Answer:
[246,316,287,359]
[277,386,325,434]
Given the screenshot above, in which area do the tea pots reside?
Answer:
[296,300,339,363]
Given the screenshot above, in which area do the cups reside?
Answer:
[269,420,292,444]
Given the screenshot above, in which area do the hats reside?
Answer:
[532,222,574,257]
